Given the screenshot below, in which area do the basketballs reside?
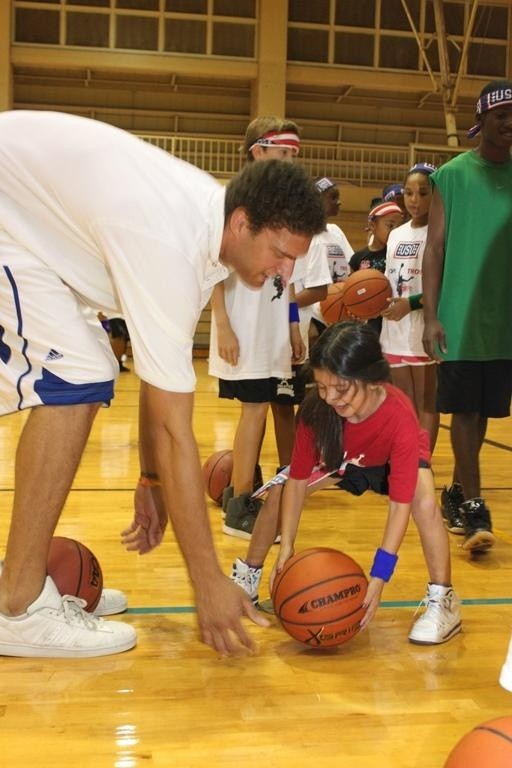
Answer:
[202,449,263,502]
[442,716,512,767]
[320,268,394,327]
[273,547,368,649]
[47,535,102,613]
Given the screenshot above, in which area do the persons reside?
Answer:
[252,235,334,493]
[0,109,328,660]
[381,183,412,224]
[109,318,131,372]
[229,319,462,646]
[207,114,307,545]
[345,162,440,456]
[348,199,404,338]
[419,79,512,555]
[308,175,355,355]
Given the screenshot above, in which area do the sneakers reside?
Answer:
[406,580,465,647]
[220,485,285,544]
[228,556,265,606]
[92,584,131,618]
[1,573,140,660]
[462,496,493,555]
[439,483,469,536]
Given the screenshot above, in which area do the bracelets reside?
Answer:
[138,471,161,488]
[369,547,398,582]
[408,293,424,311]
[289,302,300,324]
